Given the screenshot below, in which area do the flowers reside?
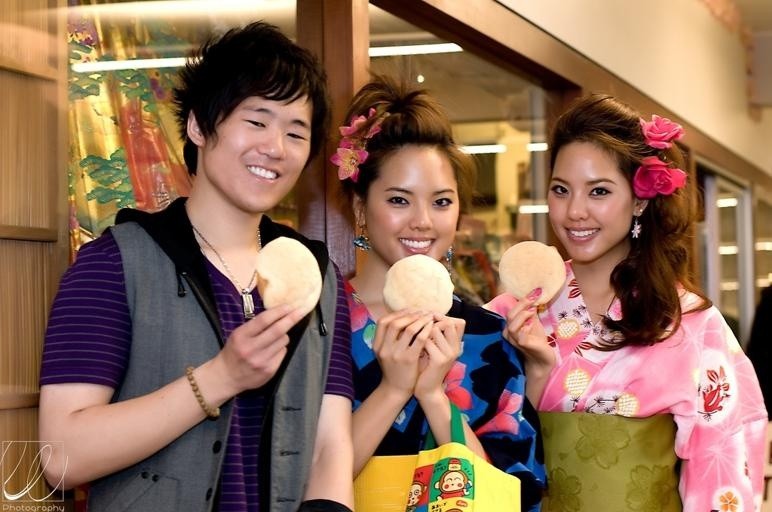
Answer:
[631,110,691,204]
[328,105,394,181]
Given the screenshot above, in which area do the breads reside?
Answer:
[498,239,568,308]
[254,235,322,317]
[382,253,455,318]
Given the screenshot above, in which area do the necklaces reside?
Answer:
[187,219,263,322]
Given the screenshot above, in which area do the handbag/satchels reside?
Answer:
[405,400,522,512]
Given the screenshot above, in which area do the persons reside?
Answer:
[326,62,550,512]
[477,91,772,512]
[35,19,359,512]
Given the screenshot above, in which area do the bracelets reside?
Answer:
[184,363,222,422]
